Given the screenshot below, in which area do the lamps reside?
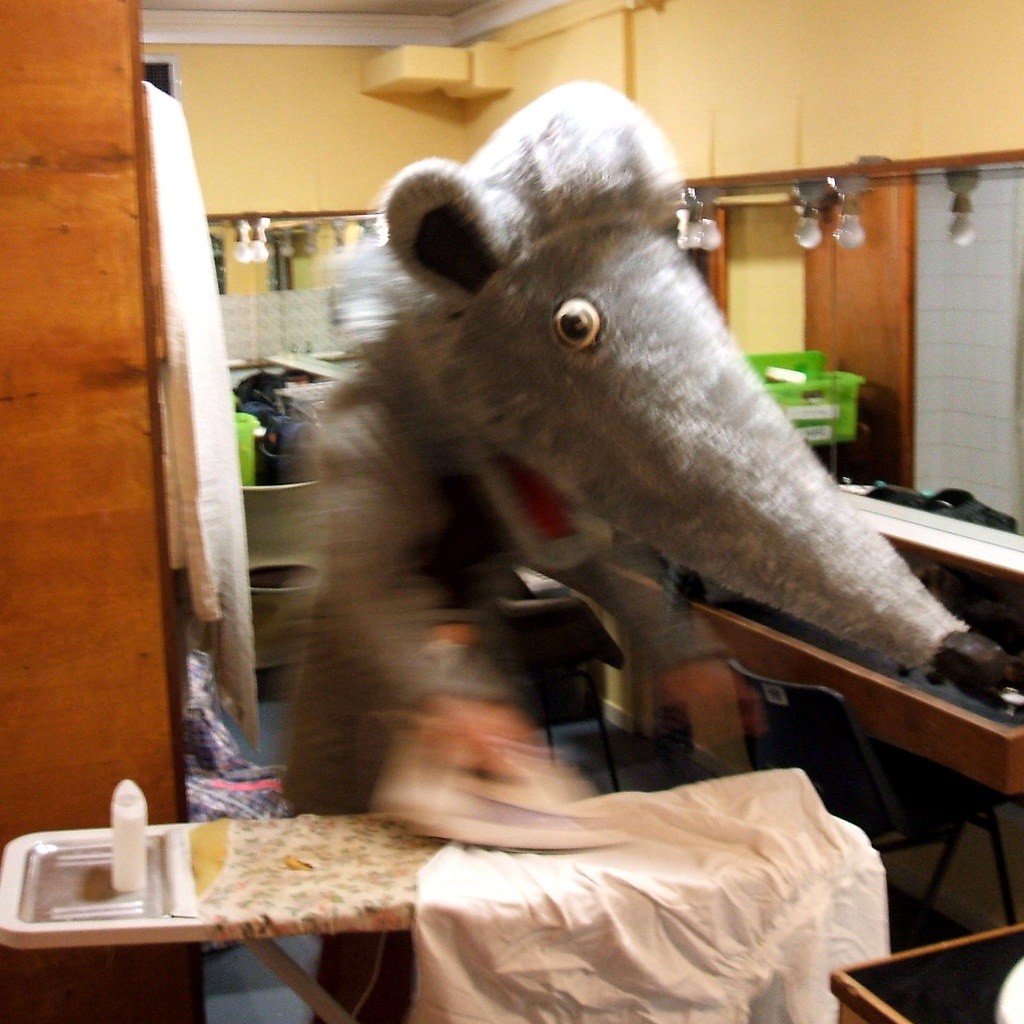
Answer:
[791,181,830,248]
[277,224,295,259]
[326,219,346,254]
[300,220,320,257]
[232,220,252,263]
[246,218,270,263]
[942,169,981,247]
[676,187,726,251]
[827,176,874,249]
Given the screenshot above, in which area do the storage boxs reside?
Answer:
[744,351,865,445]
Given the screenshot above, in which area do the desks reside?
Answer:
[521,489,1024,795]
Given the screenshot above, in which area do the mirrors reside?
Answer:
[204,212,386,389]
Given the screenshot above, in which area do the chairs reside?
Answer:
[625,517,738,794]
[724,656,1017,952]
